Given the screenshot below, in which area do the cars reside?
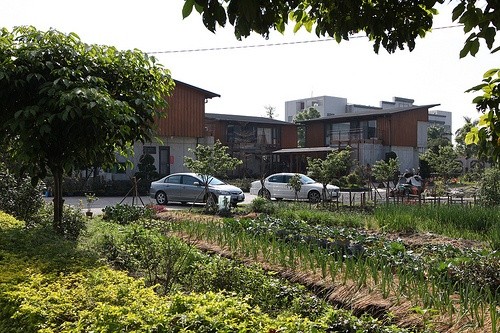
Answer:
[388,184,418,198]
[150,172,244,207]
[250,173,340,204]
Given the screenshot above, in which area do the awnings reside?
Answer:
[270,145,338,155]
